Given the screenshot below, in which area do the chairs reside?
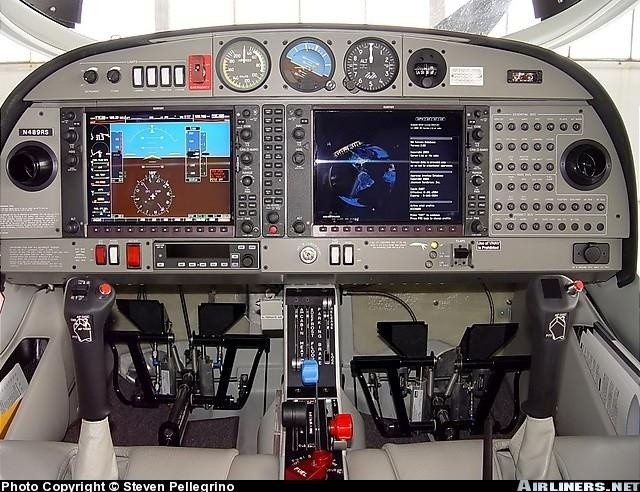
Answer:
[0,434,640,491]
